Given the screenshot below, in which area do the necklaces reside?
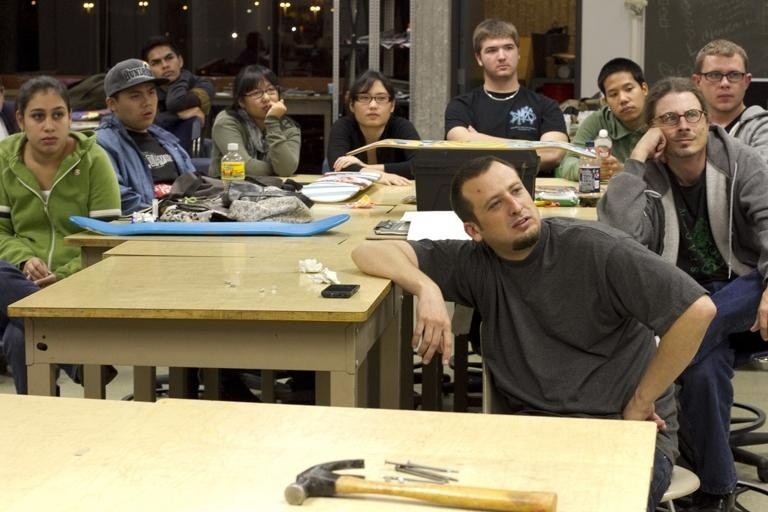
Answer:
[482,85,520,103]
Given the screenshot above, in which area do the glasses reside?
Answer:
[356,91,390,103]
[700,69,745,84]
[243,87,278,100]
[651,108,704,126]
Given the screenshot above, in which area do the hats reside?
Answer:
[104,58,169,97]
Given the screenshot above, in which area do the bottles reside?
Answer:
[578,141,601,194]
[593,129,612,165]
[220,143,244,187]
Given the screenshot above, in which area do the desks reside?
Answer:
[0,175,657,512]
[206,94,332,162]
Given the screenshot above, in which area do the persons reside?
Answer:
[690,40,768,164]
[596,77,768,512]
[0,84,21,141]
[556,58,652,182]
[351,154,717,512]
[94,58,197,214]
[141,36,213,151]
[443,17,570,175]
[0,76,122,395]
[209,62,301,180]
[326,69,422,186]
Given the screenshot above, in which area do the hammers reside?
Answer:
[285,459,557,512]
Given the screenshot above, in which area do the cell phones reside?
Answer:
[322,285,359,298]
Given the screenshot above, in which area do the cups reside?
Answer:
[327,83,333,95]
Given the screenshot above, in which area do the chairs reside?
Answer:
[479,321,700,512]
[192,117,202,160]
[678,329,768,512]
